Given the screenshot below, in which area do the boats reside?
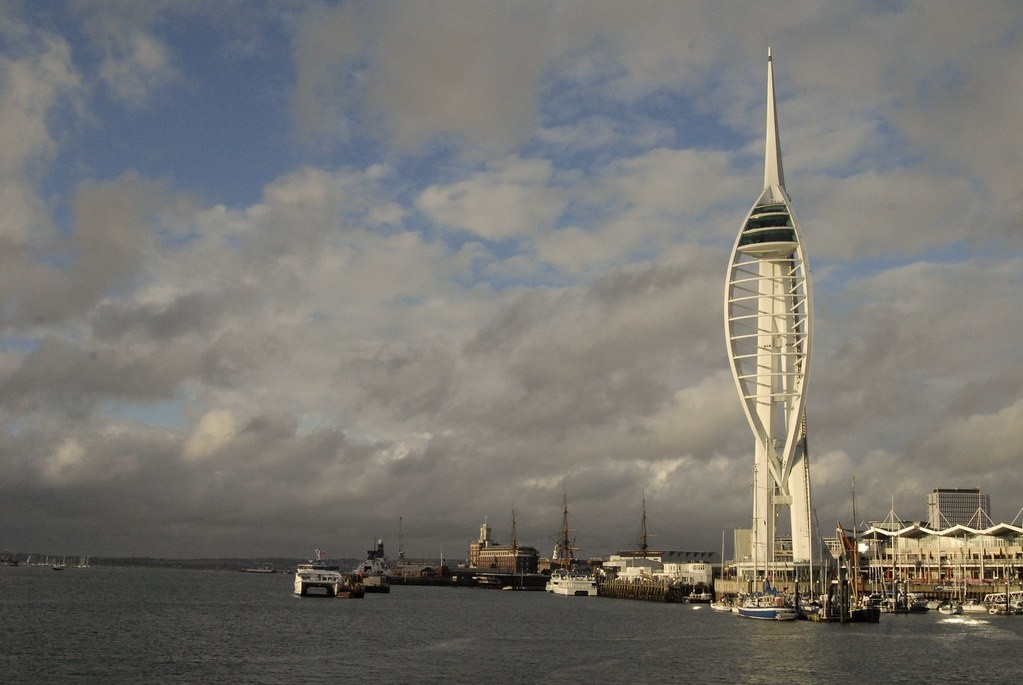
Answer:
[711,529,732,613]
[806,595,1023,622]
[681,586,712,604]
[0,554,92,571]
[735,595,798,621]
[242,562,275,574]
[294,551,342,598]
[477,577,502,588]
[336,573,367,599]
[545,568,598,596]
[353,538,390,594]
